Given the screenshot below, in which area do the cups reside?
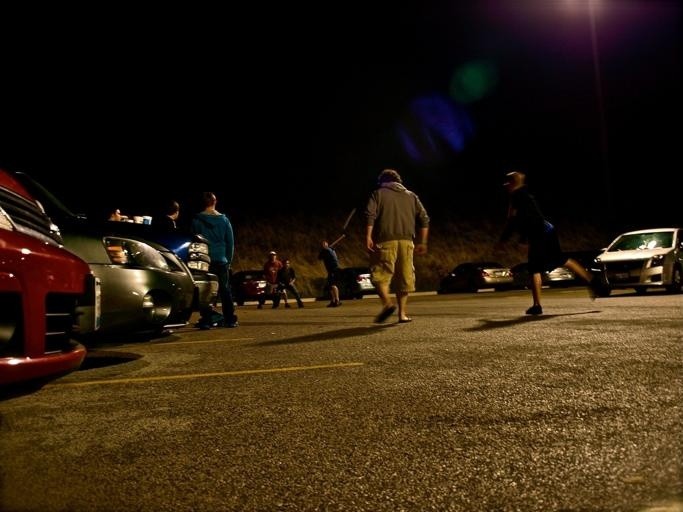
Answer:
[121,215,153,225]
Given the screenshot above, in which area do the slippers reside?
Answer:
[374,305,397,324]
[400,314,412,322]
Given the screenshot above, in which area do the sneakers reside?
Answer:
[588,276,601,301]
[255,301,345,309]
[525,303,543,317]
[197,312,238,328]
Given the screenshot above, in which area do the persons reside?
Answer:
[502,171,600,315]
[363,170,431,324]
[271,258,304,309]
[152,200,218,321]
[188,191,240,329]
[257,251,292,310]
[317,239,342,308]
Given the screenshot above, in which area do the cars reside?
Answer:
[446,260,517,286]
[230,268,275,305]
[588,227,683,295]
[509,261,576,287]
[338,265,381,301]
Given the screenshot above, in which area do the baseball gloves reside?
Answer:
[328,234,346,248]
